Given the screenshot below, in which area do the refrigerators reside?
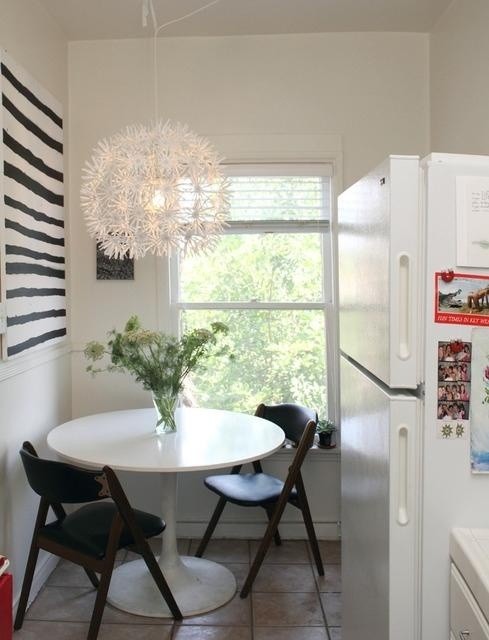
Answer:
[337,148,488,640]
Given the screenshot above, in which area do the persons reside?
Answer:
[438,344,470,420]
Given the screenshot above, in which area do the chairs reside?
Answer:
[195,402,326,599]
[13,441,184,640]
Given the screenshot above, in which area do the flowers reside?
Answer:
[82,316,231,379]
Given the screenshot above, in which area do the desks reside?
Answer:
[46,406,285,620]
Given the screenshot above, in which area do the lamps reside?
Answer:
[78,28,235,262]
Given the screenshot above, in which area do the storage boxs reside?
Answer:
[0,554,14,640]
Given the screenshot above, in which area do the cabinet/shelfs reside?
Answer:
[446,526,489,640]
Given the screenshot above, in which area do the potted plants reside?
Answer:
[316,419,338,449]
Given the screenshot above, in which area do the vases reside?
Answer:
[151,388,182,433]
